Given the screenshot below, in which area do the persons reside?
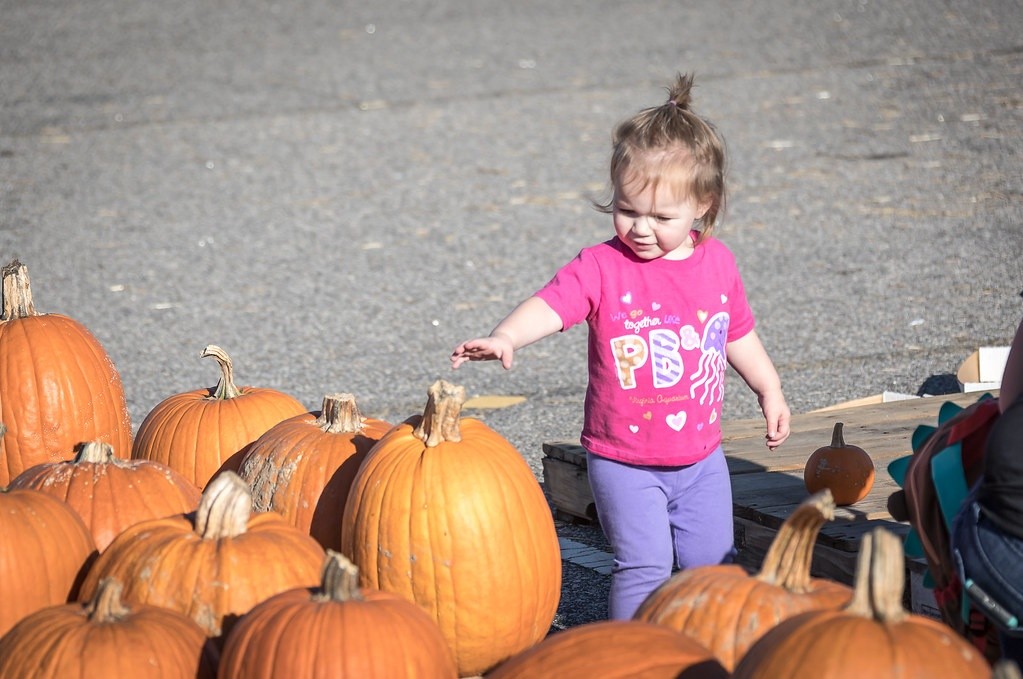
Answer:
[450,70,791,623]
[948,318,1023,679]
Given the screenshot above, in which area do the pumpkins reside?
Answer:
[0,259,998,676]
